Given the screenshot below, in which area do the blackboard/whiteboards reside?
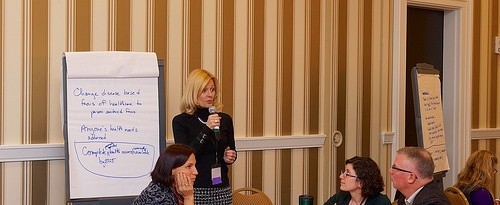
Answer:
[411,63,450,178]
[61,55,167,202]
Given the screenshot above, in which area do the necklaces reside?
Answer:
[360,195,367,205]
[197,117,207,124]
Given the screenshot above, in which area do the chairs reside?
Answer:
[444,186,469,205]
[232,188,273,205]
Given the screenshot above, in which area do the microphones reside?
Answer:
[209,106,220,141]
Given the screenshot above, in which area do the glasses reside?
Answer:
[341,169,362,180]
[392,164,418,179]
[492,167,498,175]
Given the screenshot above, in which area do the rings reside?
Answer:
[185,184,188,186]
[215,119,217,123]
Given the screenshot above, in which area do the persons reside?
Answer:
[323,155,392,205]
[172,69,237,205]
[388,147,452,205]
[453,149,498,205]
[131,144,198,205]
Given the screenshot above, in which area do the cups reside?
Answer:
[299,195,314,205]
